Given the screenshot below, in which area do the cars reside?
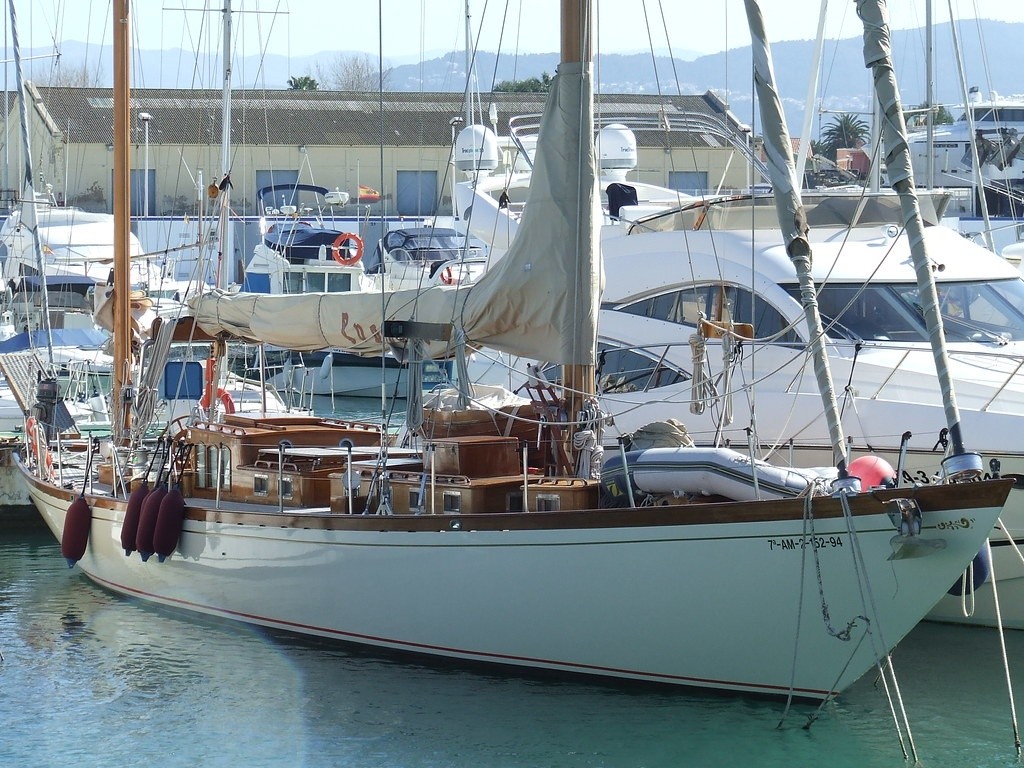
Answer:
[1,207,164,286]
[370,228,489,265]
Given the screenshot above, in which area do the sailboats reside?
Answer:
[0,0,1024,707]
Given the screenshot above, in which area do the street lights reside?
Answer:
[449,117,464,219]
[136,110,154,218]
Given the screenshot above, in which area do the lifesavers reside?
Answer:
[26,417,55,479]
[332,233,364,266]
[198,387,235,414]
[440,267,452,284]
